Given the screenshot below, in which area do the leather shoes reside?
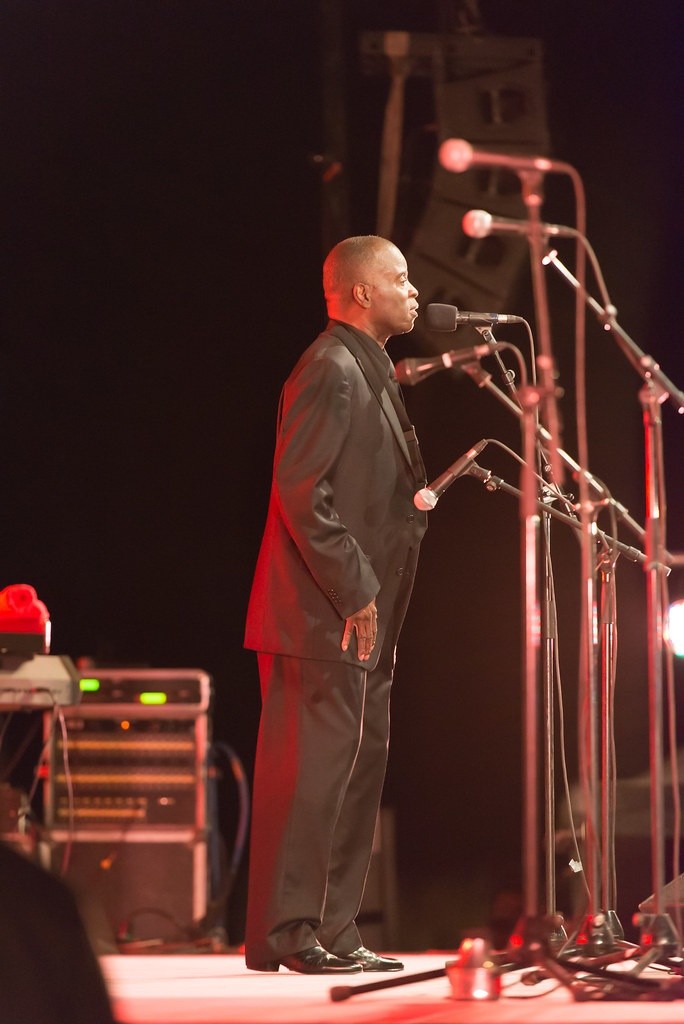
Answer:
[245,940,364,975]
[339,945,405,973]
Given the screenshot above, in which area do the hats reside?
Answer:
[0,585,51,634]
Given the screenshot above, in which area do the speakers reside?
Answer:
[392,32,552,352]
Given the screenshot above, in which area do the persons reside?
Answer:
[245,236,426,977]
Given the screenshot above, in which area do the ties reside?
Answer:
[382,351,401,394]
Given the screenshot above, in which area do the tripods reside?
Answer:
[330,176,684,1003]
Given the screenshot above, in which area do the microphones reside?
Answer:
[438,138,569,175]
[394,340,506,386]
[423,303,523,333]
[414,436,487,511]
[462,210,576,240]
